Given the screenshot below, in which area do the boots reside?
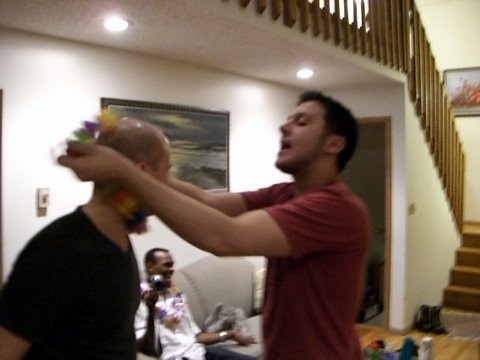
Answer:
[418,305,432,331]
[430,306,445,334]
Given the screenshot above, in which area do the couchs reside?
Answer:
[172,253,264,359]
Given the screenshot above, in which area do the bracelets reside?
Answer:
[217,331,229,342]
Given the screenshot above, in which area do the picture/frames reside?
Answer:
[100,95,231,194]
[443,67,479,120]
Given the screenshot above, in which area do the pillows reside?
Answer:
[250,268,269,315]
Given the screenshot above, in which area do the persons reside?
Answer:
[57,91,371,360]
[135,248,261,360]
[0,116,174,359]
